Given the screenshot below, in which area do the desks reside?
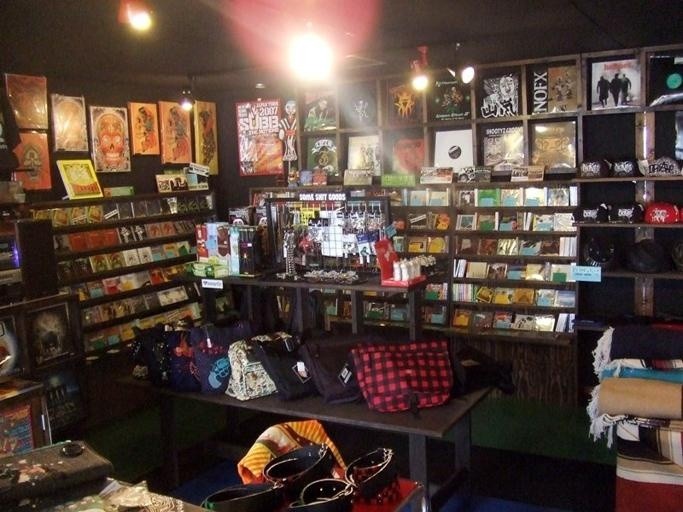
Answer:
[611,419,682,512]
[114,374,494,512]
[252,465,432,512]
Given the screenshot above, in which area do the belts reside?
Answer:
[263,443,335,491]
[345,448,396,496]
[200,484,281,512]
[288,479,355,512]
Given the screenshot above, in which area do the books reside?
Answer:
[401,188,575,335]
[33,194,216,353]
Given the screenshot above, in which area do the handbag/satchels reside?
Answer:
[252,335,318,401]
[126,334,162,380]
[225,330,300,401]
[348,339,455,414]
[297,334,386,407]
[145,332,181,387]
[191,321,255,395]
[161,328,201,392]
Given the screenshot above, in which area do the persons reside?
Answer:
[305,99,330,130]
[596,72,631,107]
[277,100,297,162]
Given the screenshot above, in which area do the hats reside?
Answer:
[610,160,644,177]
[628,239,667,273]
[647,157,682,177]
[667,236,683,273]
[572,202,611,224]
[644,203,683,225]
[582,234,618,271]
[579,159,610,178]
[608,203,644,224]
